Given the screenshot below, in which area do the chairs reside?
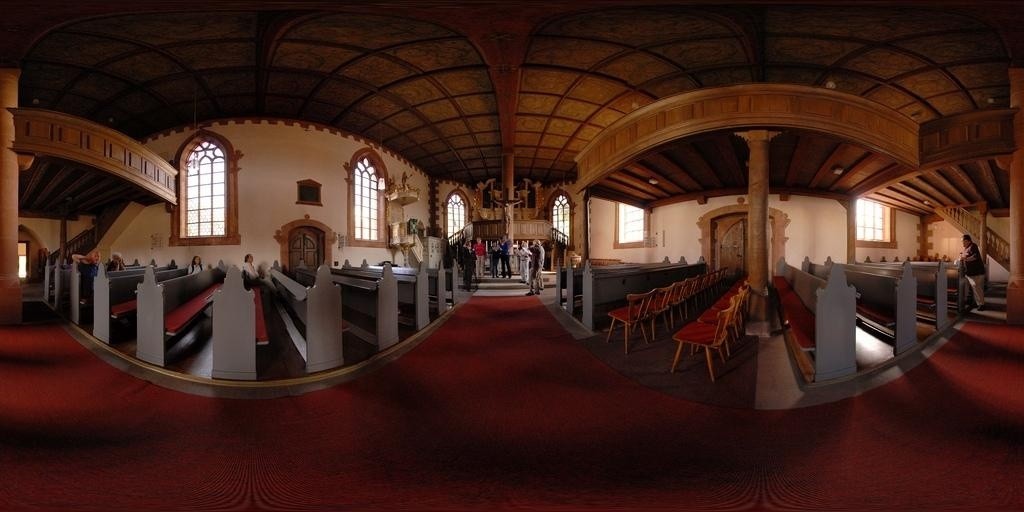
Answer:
[602,265,748,383]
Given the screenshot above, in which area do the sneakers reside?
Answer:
[527,291,541,295]
[977,305,985,311]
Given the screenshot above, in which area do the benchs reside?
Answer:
[42,254,459,384]
[769,252,971,389]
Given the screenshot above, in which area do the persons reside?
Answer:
[499,233,516,282]
[958,234,987,310]
[243,252,279,292]
[514,240,533,287]
[523,239,543,296]
[539,244,546,291]
[472,236,488,277]
[187,256,205,276]
[71,251,101,299]
[460,240,478,291]
[109,252,129,272]
[490,240,502,278]
